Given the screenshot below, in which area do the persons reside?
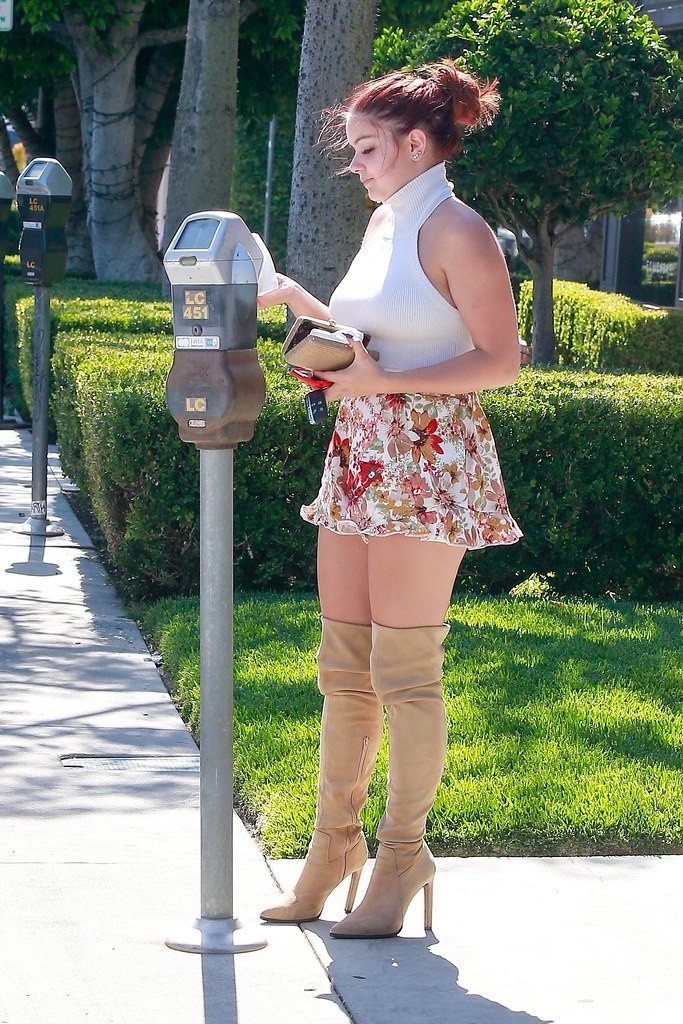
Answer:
[257,57,524,940]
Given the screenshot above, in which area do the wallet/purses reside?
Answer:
[282,315,380,371]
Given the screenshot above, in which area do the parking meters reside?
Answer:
[14,157,74,536]
[161,208,275,957]
[0,169,18,425]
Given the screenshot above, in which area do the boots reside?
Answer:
[329,620,451,939]
[260,615,383,924]
